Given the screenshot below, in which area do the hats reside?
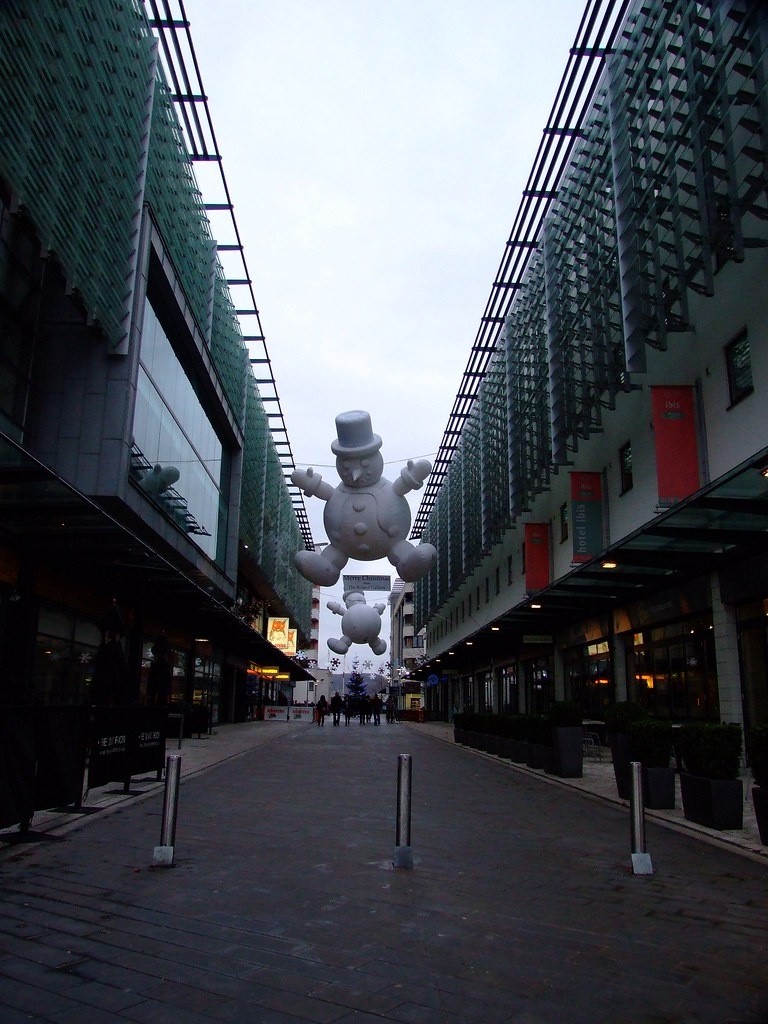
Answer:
[366,695,370,697]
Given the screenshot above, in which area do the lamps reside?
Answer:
[194,635,209,642]
[600,560,619,569]
[530,602,543,609]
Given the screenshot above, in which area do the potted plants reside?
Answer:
[632,720,676,809]
[452,711,509,759]
[748,724,768,847]
[673,723,743,831]
[525,713,548,769]
[550,701,584,778]
[510,716,525,763]
[606,701,636,800]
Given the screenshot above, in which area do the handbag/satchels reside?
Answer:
[322,703,329,714]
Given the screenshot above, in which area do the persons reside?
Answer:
[316,691,394,726]
